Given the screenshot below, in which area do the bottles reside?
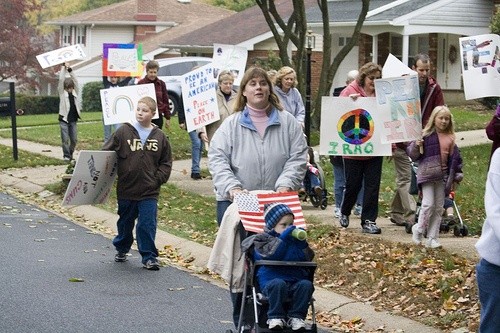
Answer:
[291,228,307,241]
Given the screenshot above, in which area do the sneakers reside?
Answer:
[142,260,160,270]
[425,237,442,250]
[114,251,128,263]
[267,319,285,329]
[287,317,307,331]
[360,220,381,234]
[339,212,350,227]
[412,224,424,245]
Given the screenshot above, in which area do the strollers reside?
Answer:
[405,156,469,236]
[225,219,318,333]
[298,147,328,210]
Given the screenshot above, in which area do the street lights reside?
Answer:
[304,29,315,147]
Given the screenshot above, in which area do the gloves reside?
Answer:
[278,226,296,242]
[296,227,307,248]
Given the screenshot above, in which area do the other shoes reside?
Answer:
[406,224,427,238]
[335,208,342,219]
[391,217,407,226]
[63,156,70,162]
[191,172,201,179]
[450,217,456,224]
[353,206,362,218]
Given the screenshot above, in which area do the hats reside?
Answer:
[263,203,295,230]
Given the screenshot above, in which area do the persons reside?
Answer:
[58,63,81,161]
[475,148,500,333]
[338,61,384,234]
[196,71,238,148]
[329,70,364,218]
[485,103,500,172]
[103,75,136,143]
[406,104,464,250]
[101,96,172,270]
[307,154,322,194]
[387,53,444,233]
[138,61,171,130]
[207,67,309,333]
[252,202,315,331]
[177,65,203,180]
[267,66,306,197]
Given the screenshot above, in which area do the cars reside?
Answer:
[127,56,214,117]
